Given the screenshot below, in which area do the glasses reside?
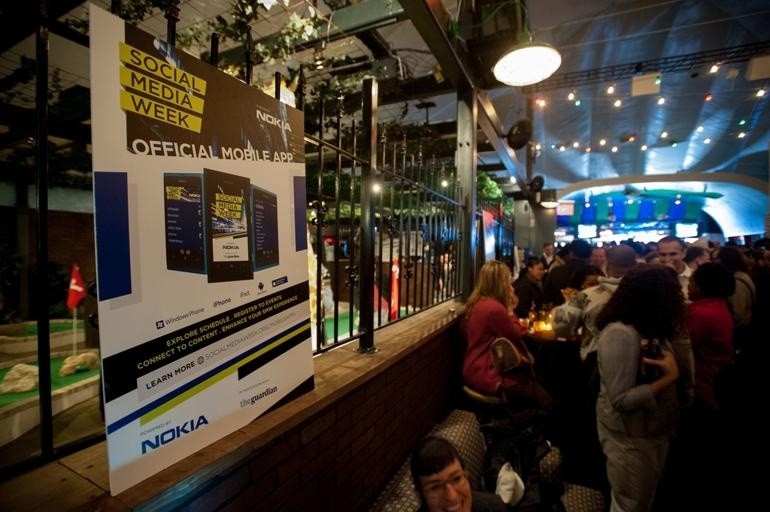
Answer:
[418,467,470,499]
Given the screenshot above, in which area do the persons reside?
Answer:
[324,236,769,512]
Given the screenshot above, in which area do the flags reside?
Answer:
[67,268,87,310]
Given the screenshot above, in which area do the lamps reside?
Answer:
[534,187,559,209]
[534,55,764,153]
[451,1,562,86]
[673,193,682,205]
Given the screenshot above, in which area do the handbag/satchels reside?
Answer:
[489,335,536,376]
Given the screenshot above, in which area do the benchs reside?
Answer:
[367,410,606,512]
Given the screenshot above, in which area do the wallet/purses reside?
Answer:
[638,345,662,382]
